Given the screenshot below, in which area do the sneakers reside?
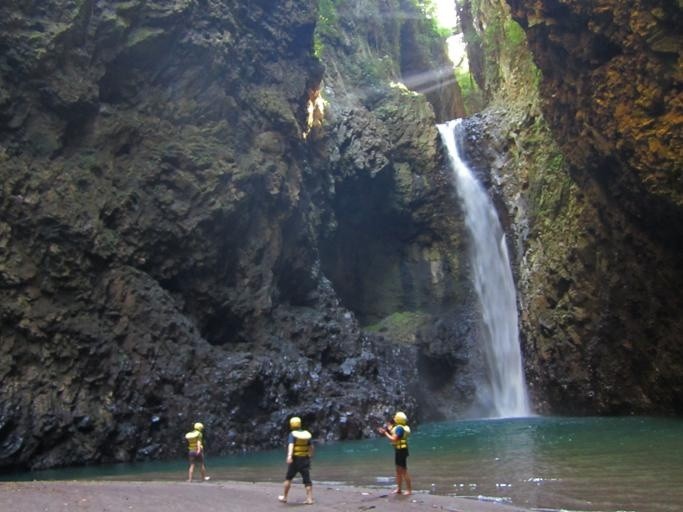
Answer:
[278,496,287,503]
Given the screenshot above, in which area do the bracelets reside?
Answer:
[388,427,392,432]
[383,430,386,436]
[285,453,293,456]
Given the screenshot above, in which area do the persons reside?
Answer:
[376,410,413,495]
[277,415,314,505]
[184,421,211,483]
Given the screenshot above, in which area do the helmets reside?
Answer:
[289,416,301,429]
[194,423,204,431]
[393,412,407,426]
